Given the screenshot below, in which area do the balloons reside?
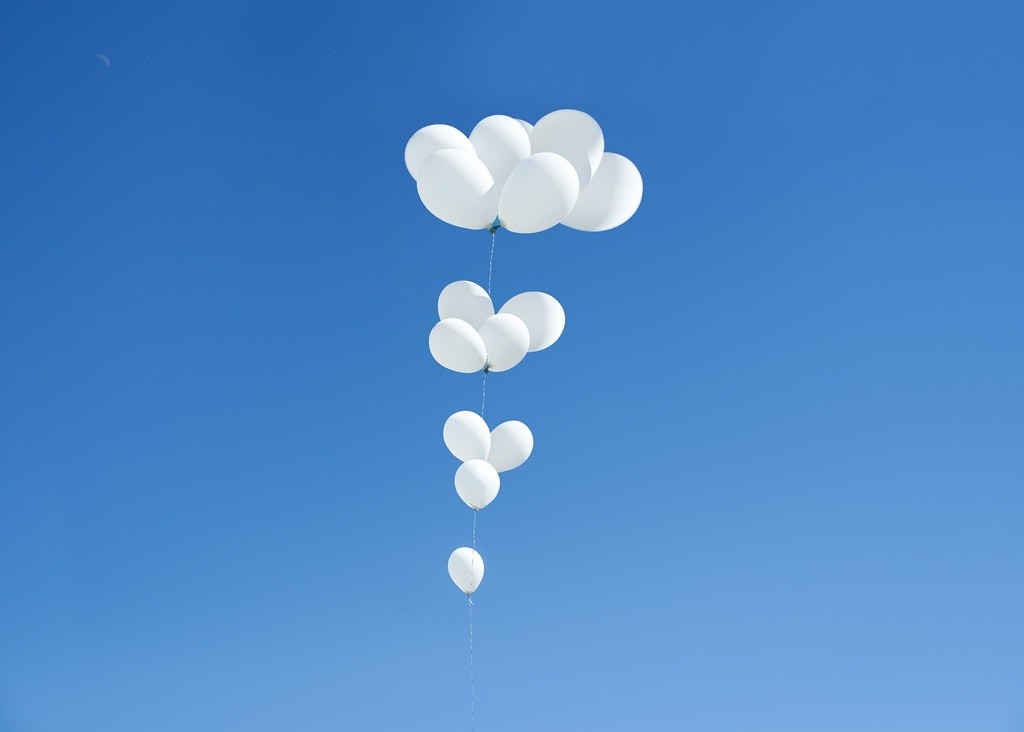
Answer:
[437,279,495,332]
[498,150,580,234]
[416,147,500,231]
[469,114,532,198]
[514,117,535,143]
[496,291,566,353]
[476,312,530,373]
[488,419,534,473]
[529,109,605,193]
[559,150,644,233]
[442,410,491,462]
[403,123,479,182]
[454,458,501,511]
[428,318,487,374]
[447,546,485,596]
